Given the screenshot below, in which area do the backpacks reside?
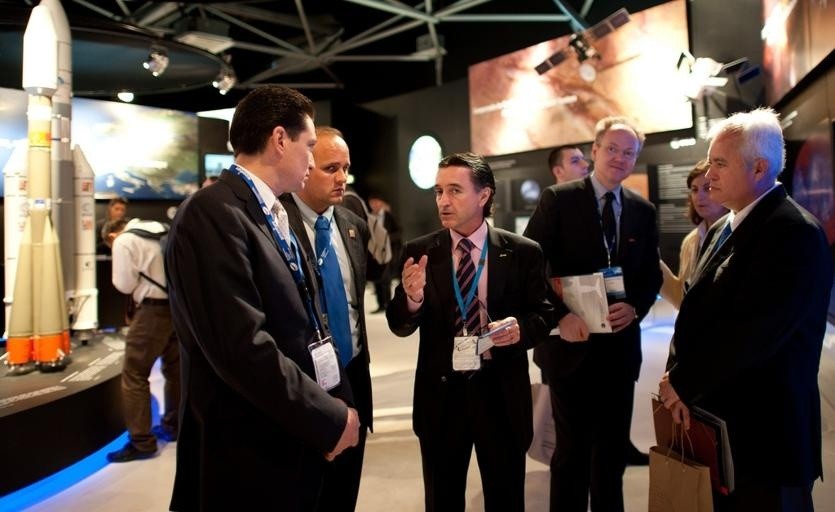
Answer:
[126,220,171,291]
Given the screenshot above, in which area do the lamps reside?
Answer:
[207,58,243,102]
[141,47,171,81]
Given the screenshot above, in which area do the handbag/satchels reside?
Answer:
[649,446,714,512]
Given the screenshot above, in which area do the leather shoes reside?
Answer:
[152,425,177,442]
[107,447,157,462]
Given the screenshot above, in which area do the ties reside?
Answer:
[315,216,352,369]
[701,223,731,272]
[602,192,617,267]
[455,239,481,380]
[273,199,291,248]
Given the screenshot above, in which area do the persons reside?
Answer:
[546,145,591,186]
[518,114,664,510]
[94,197,128,251]
[272,127,374,510]
[167,81,362,511]
[201,174,220,187]
[364,191,397,314]
[657,109,834,511]
[386,152,555,511]
[98,215,182,461]
[651,156,732,311]
